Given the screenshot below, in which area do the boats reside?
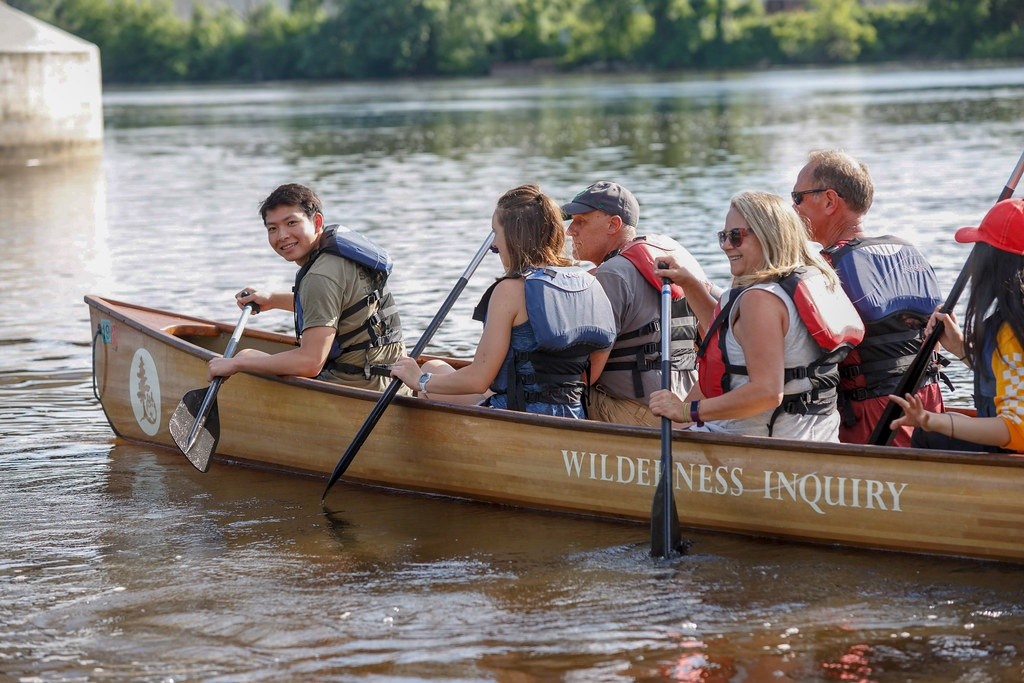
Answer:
[79,295,1023,618]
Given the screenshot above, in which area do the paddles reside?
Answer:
[318,231,496,501]
[167,288,262,477]
[650,263,682,564]
[866,152,1024,445]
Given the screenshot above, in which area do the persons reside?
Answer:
[888,197,1024,453]
[207,182,407,391]
[649,191,866,444]
[560,180,711,429]
[792,151,955,447]
[389,184,617,419]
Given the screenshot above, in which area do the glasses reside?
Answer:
[718,227,753,250]
[792,189,843,205]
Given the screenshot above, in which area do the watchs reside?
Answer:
[690,399,704,427]
[419,373,433,393]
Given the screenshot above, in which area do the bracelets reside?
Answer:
[959,356,966,361]
[684,402,689,423]
[948,414,953,439]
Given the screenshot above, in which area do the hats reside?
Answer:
[955,197,1024,255]
[560,181,639,229]
